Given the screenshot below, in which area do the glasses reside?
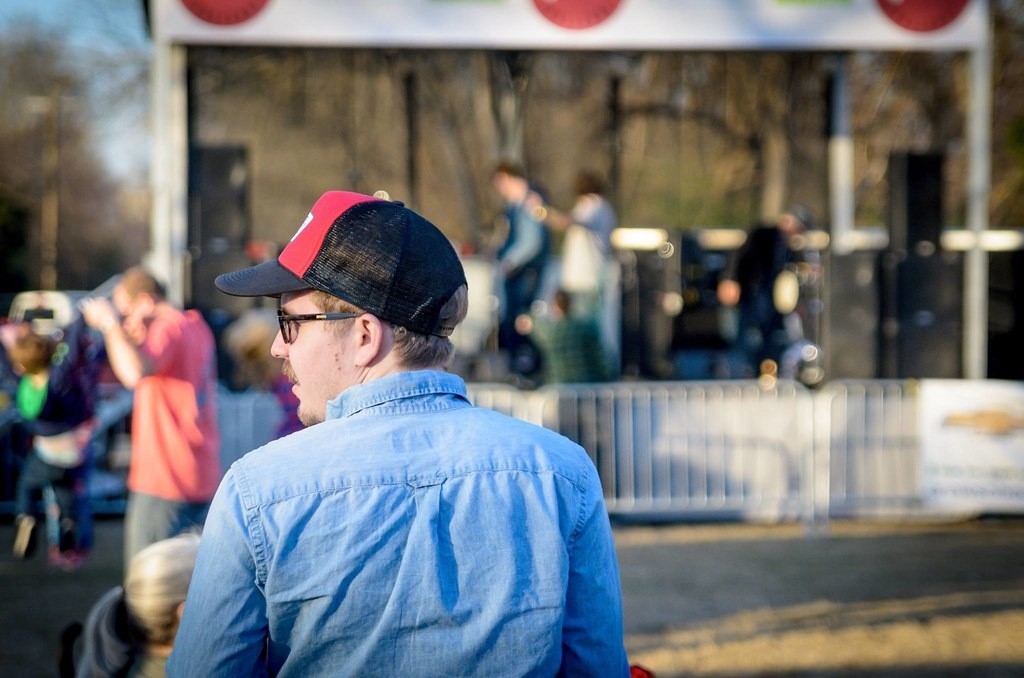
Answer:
[275,308,366,344]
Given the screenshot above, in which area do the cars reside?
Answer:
[0,274,158,520]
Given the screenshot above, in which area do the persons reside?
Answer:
[77,532,203,678]
[0,264,219,587]
[716,204,817,382]
[157,187,629,678]
[486,159,629,391]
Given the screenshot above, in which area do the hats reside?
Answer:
[219,189,471,338]
